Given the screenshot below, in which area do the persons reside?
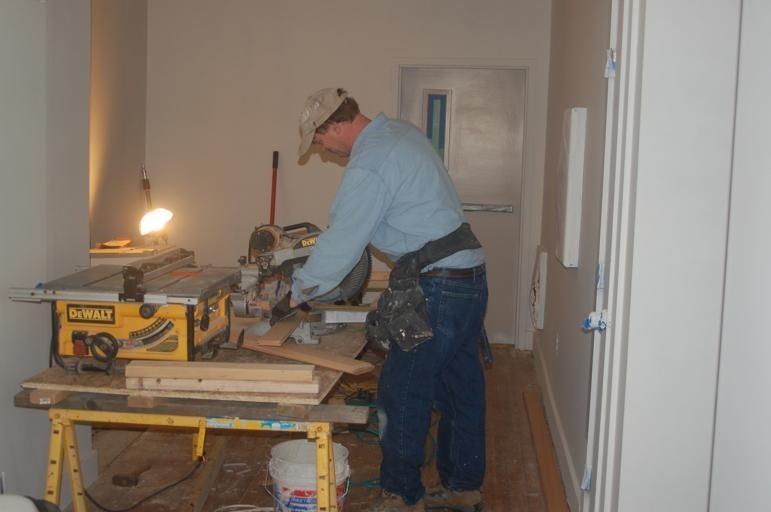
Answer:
[261,84,489,511]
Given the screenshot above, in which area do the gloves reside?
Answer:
[265,290,310,324]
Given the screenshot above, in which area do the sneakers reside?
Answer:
[362,490,424,512]
[426,483,481,509]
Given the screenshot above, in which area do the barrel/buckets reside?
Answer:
[264,439,351,512]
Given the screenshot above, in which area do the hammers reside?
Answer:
[111,463,151,487]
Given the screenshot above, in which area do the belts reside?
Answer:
[428,265,486,280]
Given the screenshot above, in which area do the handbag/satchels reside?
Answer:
[364,258,434,358]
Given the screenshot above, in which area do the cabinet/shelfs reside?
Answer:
[89,244,176,269]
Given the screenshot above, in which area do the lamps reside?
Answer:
[139,207,173,247]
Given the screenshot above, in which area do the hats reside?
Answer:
[295,88,348,161]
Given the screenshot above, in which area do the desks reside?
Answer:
[15,304,379,512]
